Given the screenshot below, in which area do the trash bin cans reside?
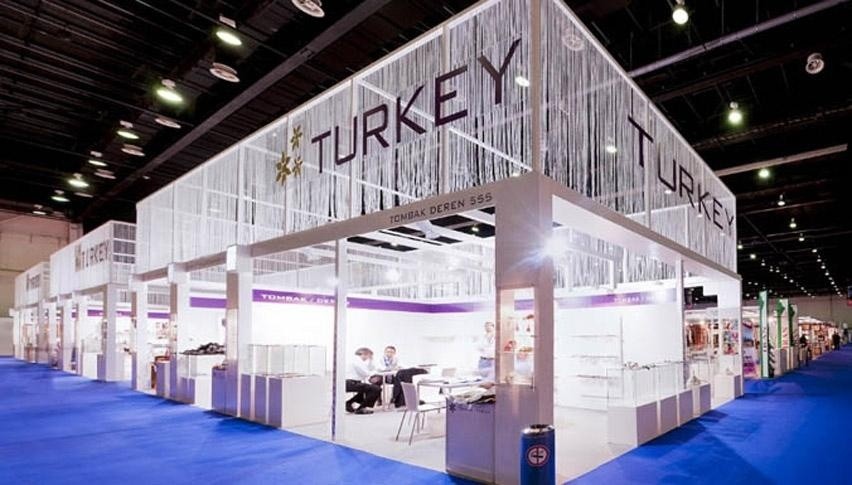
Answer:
[521,423,555,485]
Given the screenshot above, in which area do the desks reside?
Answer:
[366,368,401,413]
[412,373,483,430]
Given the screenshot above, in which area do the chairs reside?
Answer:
[393,382,447,445]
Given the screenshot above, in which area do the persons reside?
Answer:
[379,345,401,373]
[831,330,850,351]
[800,333,813,353]
[478,321,497,370]
[342,347,382,414]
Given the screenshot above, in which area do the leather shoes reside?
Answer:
[346,401,373,413]
